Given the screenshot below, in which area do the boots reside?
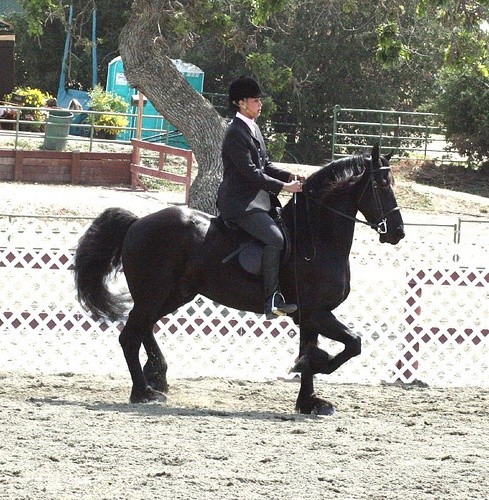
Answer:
[262,244,298,321]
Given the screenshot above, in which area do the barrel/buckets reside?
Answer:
[43,109,74,150]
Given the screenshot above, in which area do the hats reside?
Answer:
[229,76,268,104]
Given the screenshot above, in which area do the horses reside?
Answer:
[74,142,405,416]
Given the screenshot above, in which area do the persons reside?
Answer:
[215,76,307,319]
